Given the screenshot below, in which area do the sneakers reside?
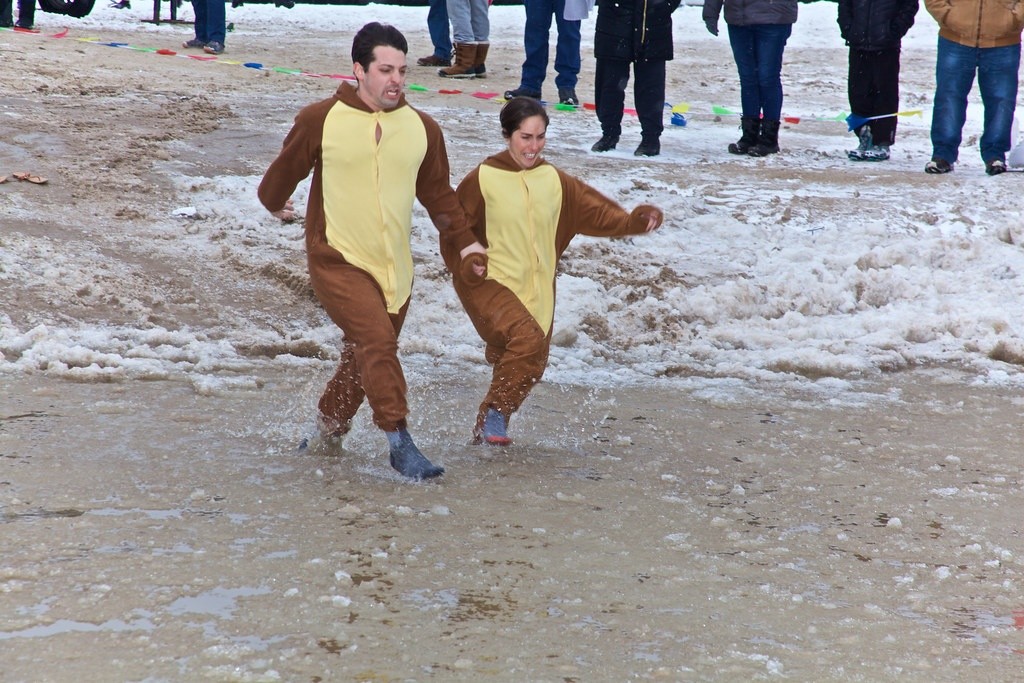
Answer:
[865,144,890,159]
[926,159,953,172]
[986,160,1007,173]
[504,88,541,100]
[204,41,225,53]
[558,87,579,106]
[849,141,872,158]
[183,38,206,49]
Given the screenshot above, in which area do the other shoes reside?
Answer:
[591,130,619,152]
[484,408,511,444]
[14,21,41,32]
[634,135,661,157]
[417,55,450,66]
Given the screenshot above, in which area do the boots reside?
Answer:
[474,42,490,75]
[440,43,476,77]
[748,118,780,155]
[728,115,762,152]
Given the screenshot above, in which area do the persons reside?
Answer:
[504,0,595,104]
[923,0,1024,176]
[257,22,488,479]
[827,0,919,160]
[182,0,226,54]
[439,96,663,445]
[591,0,681,156]
[0,0,36,29]
[416,0,493,78]
[702,0,799,156]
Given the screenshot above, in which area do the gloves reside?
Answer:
[705,17,719,36]
